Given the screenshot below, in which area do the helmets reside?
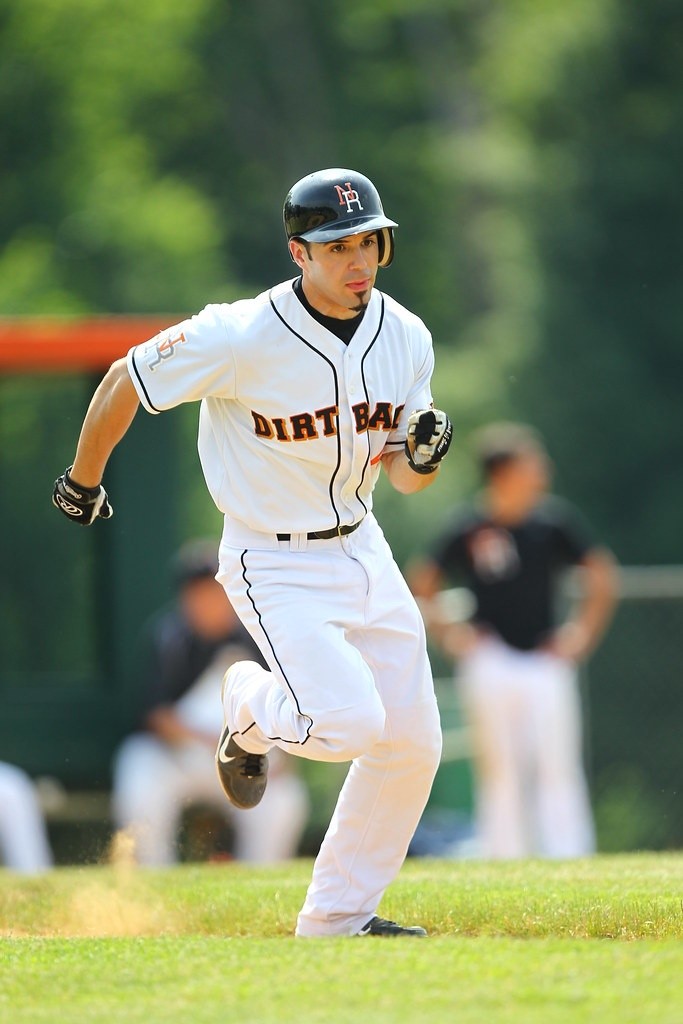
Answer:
[284,167,399,268]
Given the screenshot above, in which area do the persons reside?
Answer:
[0,761,54,870]
[111,547,310,867]
[54,168,452,935]
[411,425,618,859]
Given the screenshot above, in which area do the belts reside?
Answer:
[277,516,366,542]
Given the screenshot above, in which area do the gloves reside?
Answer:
[405,408,452,475]
[52,464,113,526]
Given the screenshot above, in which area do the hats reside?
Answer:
[157,539,223,592]
[464,415,534,486]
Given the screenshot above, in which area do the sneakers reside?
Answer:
[216,660,271,812]
[356,916,427,938]
